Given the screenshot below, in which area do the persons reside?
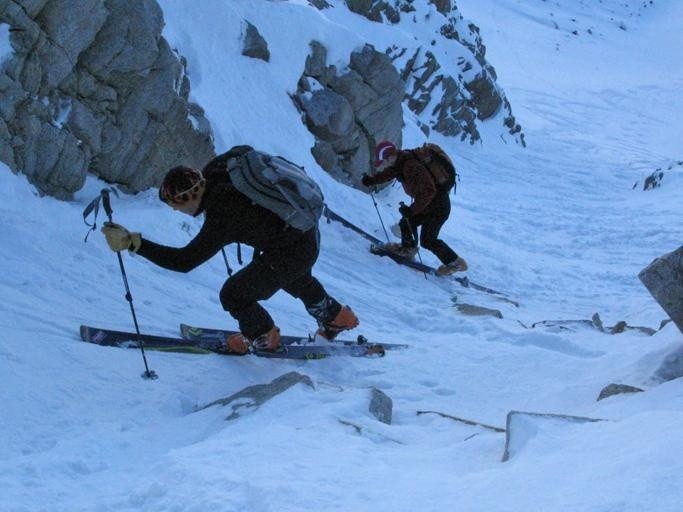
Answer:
[357,141,469,276]
[99,142,360,355]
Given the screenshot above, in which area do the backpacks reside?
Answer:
[223,151,324,233]
[411,143,456,193]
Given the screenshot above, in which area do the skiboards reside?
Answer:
[324,207,511,297]
[80,325,408,359]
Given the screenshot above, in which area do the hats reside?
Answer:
[374,142,395,167]
[159,164,204,209]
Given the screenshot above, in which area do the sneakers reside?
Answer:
[314,306,358,342]
[388,243,417,260]
[227,325,281,353]
[436,258,468,275]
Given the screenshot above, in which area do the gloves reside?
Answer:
[101,222,142,253]
[399,205,411,216]
[363,175,374,186]
